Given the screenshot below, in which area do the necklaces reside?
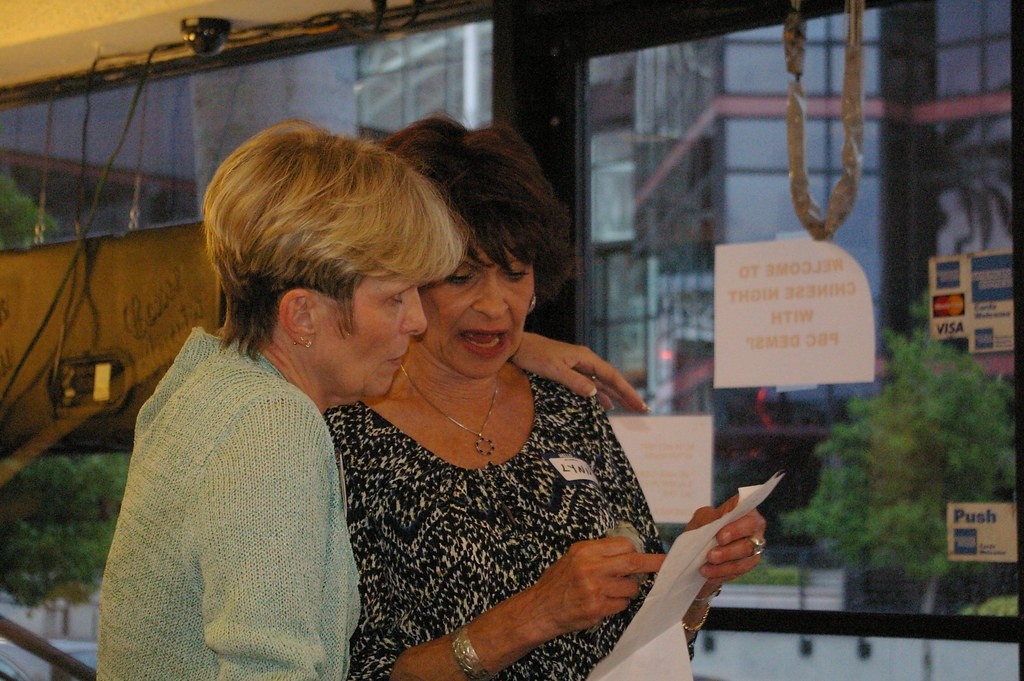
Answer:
[399,354,501,456]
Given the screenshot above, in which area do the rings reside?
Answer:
[748,536,763,557]
[591,376,595,382]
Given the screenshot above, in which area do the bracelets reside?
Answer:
[682,602,710,632]
[450,621,498,681]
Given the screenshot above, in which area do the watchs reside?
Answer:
[692,582,724,607]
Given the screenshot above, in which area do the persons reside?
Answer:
[320,114,768,681]
[92,117,651,680]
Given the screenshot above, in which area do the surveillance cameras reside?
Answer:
[181,18,231,57]
